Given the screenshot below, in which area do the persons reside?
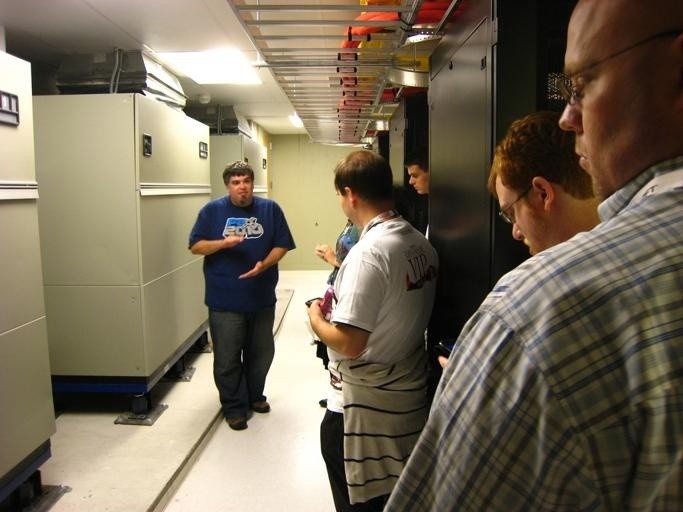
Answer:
[306,151,439,512]
[312,218,361,408]
[380,0,683,512]
[404,150,429,240]
[188,161,296,431]
[487,110,600,255]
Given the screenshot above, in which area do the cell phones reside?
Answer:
[422,327,457,354]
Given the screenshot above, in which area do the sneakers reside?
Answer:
[250,398,270,414]
[226,411,249,430]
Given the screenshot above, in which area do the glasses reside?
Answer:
[498,186,533,224]
[558,28,682,107]
